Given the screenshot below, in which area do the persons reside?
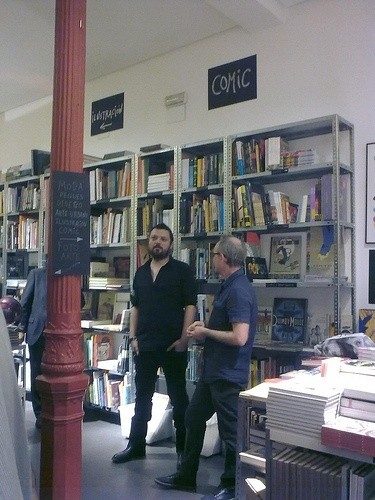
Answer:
[18,267,48,428]
[112,224,197,464]
[154,237,258,500]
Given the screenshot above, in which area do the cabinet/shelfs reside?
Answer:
[0,114,375,500]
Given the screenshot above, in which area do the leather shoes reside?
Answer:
[113,443,145,460]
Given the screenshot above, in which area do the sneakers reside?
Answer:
[211,484,235,499]
[156,472,195,491]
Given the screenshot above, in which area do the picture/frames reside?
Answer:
[364,142,375,244]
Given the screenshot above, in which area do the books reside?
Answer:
[87,136,346,412]
[0,182,37,251]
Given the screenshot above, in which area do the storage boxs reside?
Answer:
[116,402,173,445]
[201,416,221,458]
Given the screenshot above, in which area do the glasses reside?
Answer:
[213,253,221,258]
